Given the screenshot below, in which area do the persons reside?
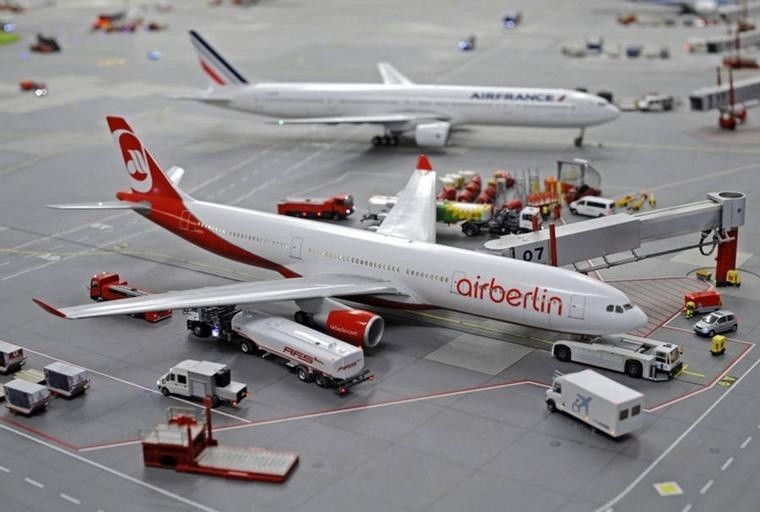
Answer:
[543,204,551,219]
[685,296,696,319]
[552,200,561,220]
